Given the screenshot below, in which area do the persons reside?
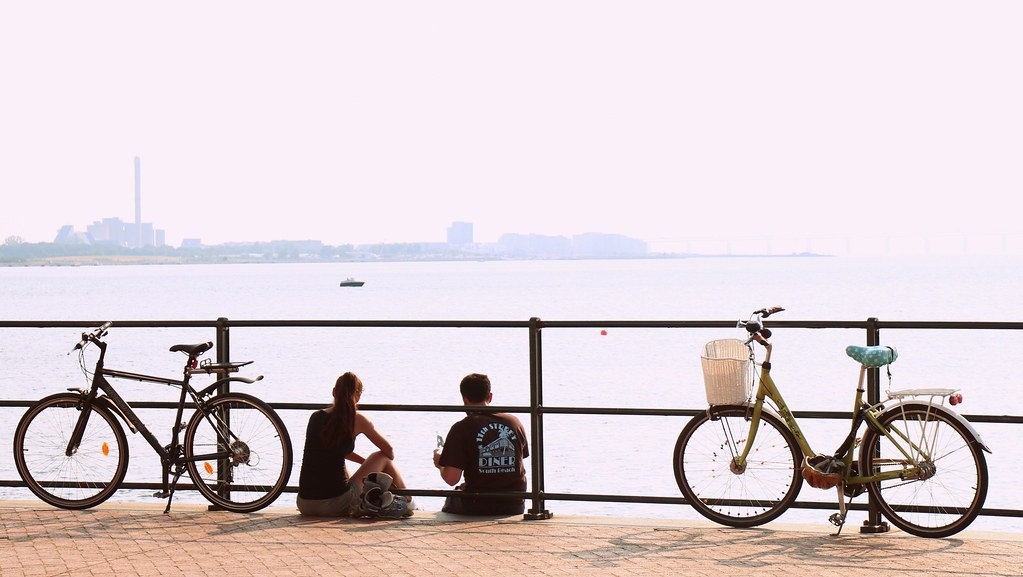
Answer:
[432,373,530,516]
[295,372,425,516]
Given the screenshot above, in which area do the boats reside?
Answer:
[339,277,364,288]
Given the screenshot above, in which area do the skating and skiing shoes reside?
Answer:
[351,471,414,520]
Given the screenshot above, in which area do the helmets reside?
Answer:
[836,461,867,498]
[800,454,841,490]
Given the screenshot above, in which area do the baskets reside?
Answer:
[698,338,756,407]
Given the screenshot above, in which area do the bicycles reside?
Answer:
[671,306,992,540]
[11,319,295,516]
